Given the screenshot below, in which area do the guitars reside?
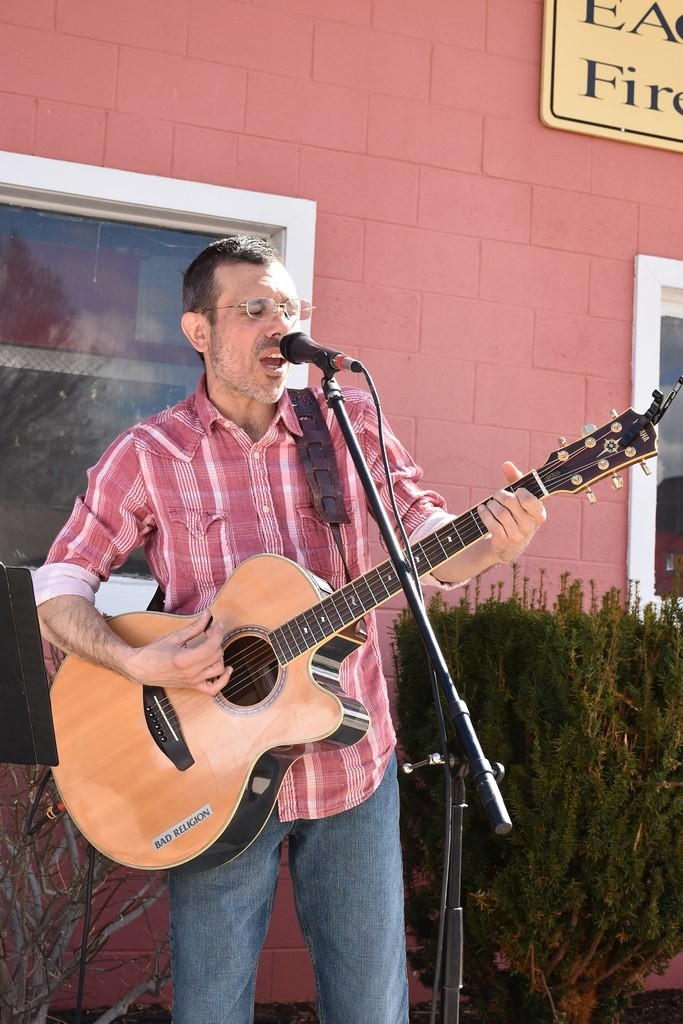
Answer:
[45,376,683,874]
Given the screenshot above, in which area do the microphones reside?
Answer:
[280,332,363,373]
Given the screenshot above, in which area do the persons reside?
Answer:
[31,233,546,1024]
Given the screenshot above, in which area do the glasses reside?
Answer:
[188,297,316,322]
